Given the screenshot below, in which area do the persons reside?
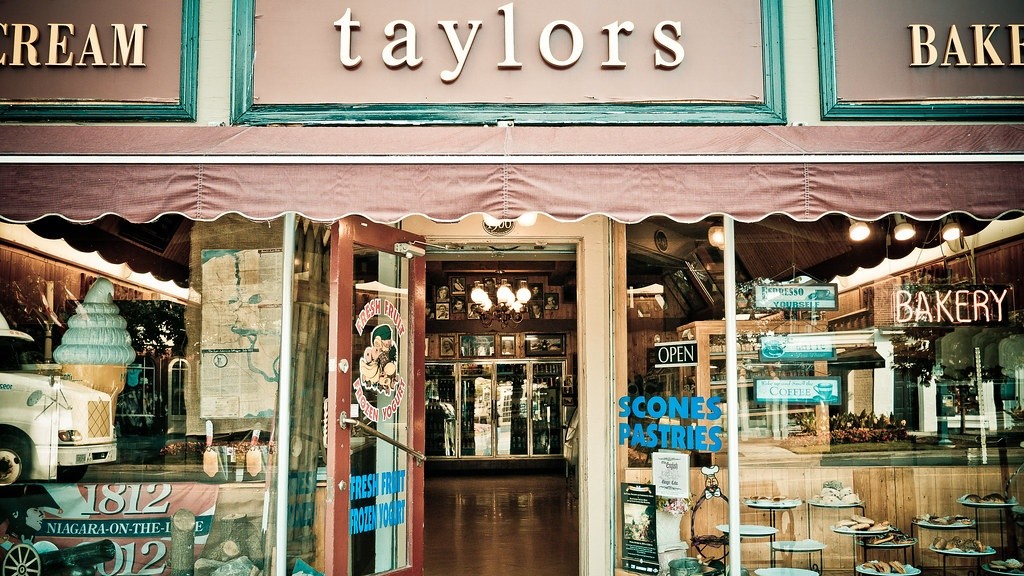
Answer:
[443,337,453,355]
[425,302,434,319]
[502,339,514,354]
[484,278,495,293]
[452,297,465,312]
[531,284,542,299]
[437,306,448,319]
[544,295,559,309]
[436,286,449,302]
[469,305,480,318]
[531,303,542,318]
[453,278,464,292]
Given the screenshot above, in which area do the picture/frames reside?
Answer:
[440,335,455,357]
[425,274,577,321]
[500,334,516,356]
[425,336,429,357]
[525,333,567,357]
[456,332,497,359]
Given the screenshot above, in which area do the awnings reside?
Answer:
[1,118,1024,226]
[718,213,1024,284]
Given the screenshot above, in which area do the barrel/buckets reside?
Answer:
[667,557,702,576]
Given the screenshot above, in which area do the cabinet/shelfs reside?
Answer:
[676,318,830,457]
[715,496,1024,575]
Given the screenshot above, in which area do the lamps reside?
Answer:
[708,218,726,248]
[892,214,915,241]
[847,219,870,243]
[469,261,532,328]
[941,217,963,242]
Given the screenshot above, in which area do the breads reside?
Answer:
[964,493,1007,504]
[856,527,915,544]
[816,480,858,504]
[748,495,800,504]
[912,512,972,525]
[988,559,1024,572]
[835,514,889,531]
[933,536,985,553]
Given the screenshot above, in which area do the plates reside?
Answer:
[753,567,820,576]
[955,498,1021,508]
[762,348,785,359]
[928,542,996,555]
[831,526,890,535]
[807,499,866,508]
[855,565,921,576]
[915,521,975,529]
[981,562,1024,576]
[856,541,919,549]
[715,523,780,537]
[761,345,785,360]
[768,540,828,552]
[812,395,839,404]
[808,294,835,300]
[744,499,800,508]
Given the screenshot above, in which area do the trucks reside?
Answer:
[0,313,119,490]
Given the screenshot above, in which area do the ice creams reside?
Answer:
[55,277,136,441]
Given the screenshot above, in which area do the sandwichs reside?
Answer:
[862,559,906,574]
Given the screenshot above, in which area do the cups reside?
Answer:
[813,381,834,401]
[813,288,831,299]
